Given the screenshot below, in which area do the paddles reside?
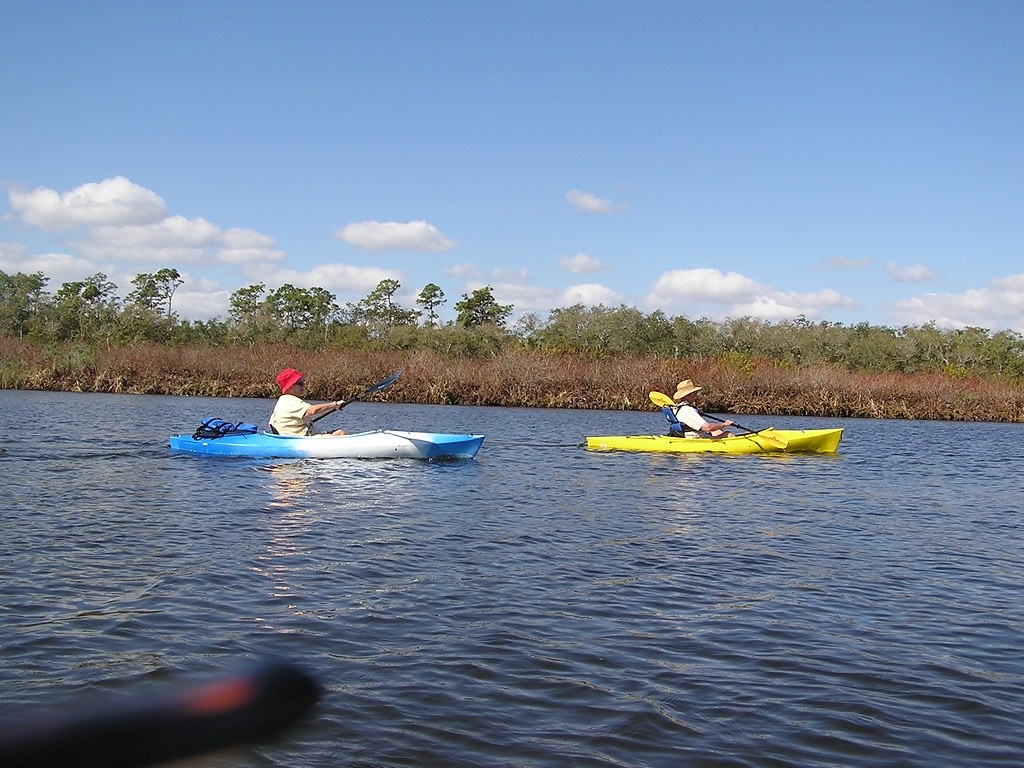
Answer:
[648,391,789,449]
[312,368,404,424]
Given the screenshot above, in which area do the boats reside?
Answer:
[0,664,325,767]
[584,426,845,453]
[169,428,487,460]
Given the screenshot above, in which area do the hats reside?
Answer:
[275,367,303,394]
[673,380,703,400]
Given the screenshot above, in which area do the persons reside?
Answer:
[269,369,347,435]
[670,380,736,438]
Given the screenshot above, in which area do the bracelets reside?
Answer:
[334,401,337,407]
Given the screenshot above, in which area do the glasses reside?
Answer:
[293,381,304,386]
